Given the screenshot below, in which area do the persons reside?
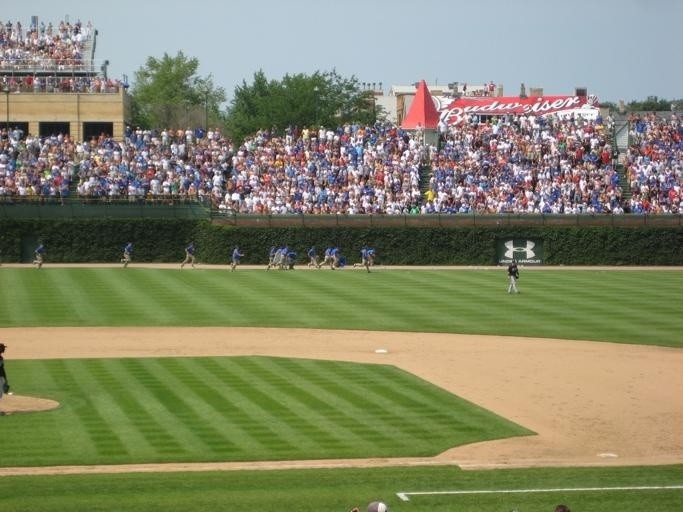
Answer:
[488,80,497,96]
[507,259,521,295]
[230,243,244,272]
[265,245,377,273]
[180,241,196,269]
[520,82,527,98]
[120,242,134,268]
[0,18,121,94]
[483,83,488,96]
[2,112,682,215]
[462,82,467,96]
[0,344,13,416]
[32,243,48,269]
